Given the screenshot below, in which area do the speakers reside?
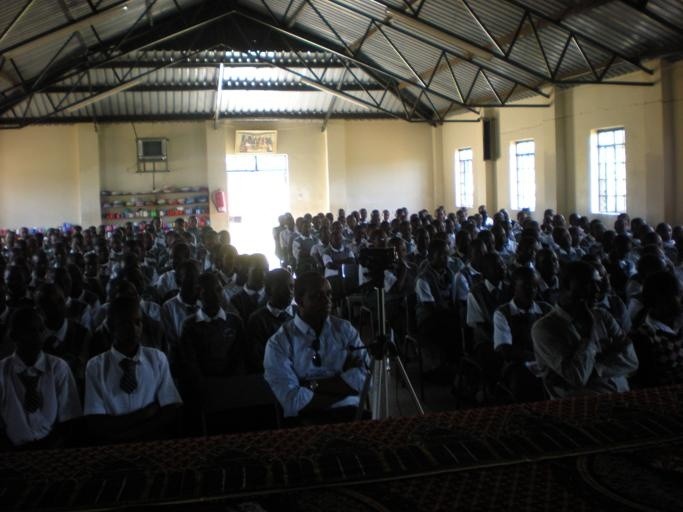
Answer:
[483,118,491,160]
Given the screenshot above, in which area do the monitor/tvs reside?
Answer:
[138,138,167,160]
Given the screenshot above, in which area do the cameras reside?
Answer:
[335,246,409,272]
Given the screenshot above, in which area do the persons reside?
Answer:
[0,205,682,451]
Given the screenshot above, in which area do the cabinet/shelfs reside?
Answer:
[100,187,210,228]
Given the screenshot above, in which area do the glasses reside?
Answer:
[311,340,322,369]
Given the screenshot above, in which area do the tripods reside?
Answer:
[349,272,425,420]
